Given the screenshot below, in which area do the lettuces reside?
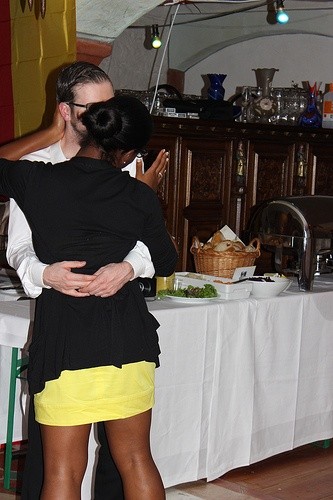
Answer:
[157,283,218,298]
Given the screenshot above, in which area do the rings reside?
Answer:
[158,173,163,178]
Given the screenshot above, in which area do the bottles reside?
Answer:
[251,68,280,123]
[322,83,333,128]
[297,92,322,128]
[207,74,227,101]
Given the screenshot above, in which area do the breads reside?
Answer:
[203,230,246,252]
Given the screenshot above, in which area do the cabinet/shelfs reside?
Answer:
[143,116,333,274]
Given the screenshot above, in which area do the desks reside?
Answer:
[0,271,333,500]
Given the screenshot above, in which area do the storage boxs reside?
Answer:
[175,271,253,301]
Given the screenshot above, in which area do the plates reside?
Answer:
[174,271,253,300]
[163,293,221,303]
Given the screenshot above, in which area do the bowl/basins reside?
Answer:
[239,276,290,297]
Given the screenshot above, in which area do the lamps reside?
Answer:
[274,0,292,25]
[149,23,162,49]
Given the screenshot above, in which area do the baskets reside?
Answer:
[190,236,261,279]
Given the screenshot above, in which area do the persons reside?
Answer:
[6,61,179,500]
[0,94,170,500]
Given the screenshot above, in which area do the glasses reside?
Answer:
[70,103,93,111]
[136,149,149,158]
